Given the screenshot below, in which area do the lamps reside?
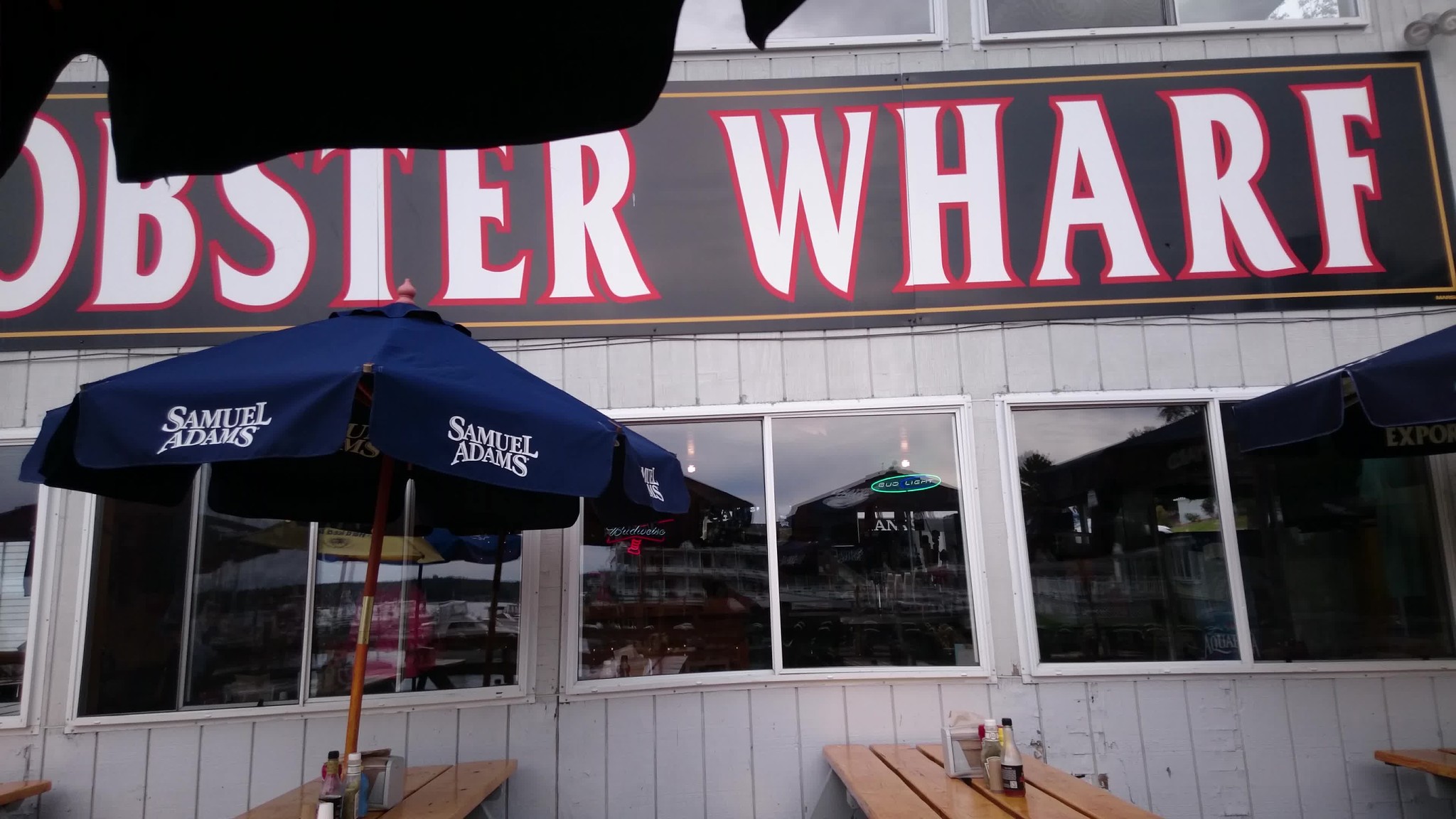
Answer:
[1404,7,1456,46]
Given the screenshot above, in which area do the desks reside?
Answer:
[224,654,466,704]
[585,651,691,680]
[0,778,52,814]
[1374,746,1456,780]
[823,744,1166,819]
[233,757,518,819]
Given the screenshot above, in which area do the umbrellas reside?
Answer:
[20,277,691,776]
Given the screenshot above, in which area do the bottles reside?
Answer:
[601,655,631,679]
[977,718,1026,798]
[341,752,370,819]
[316,751,346,819]
